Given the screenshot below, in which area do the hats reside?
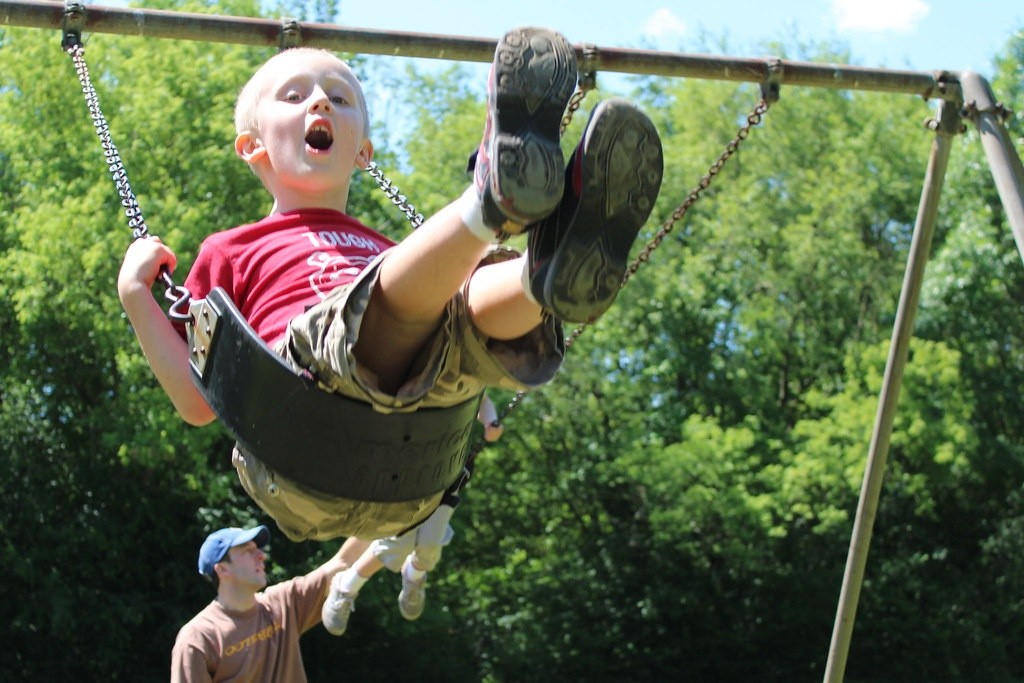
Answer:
[198,525,270,582]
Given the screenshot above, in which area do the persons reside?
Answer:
[320,391,505,636]
[117,24,663,539]
[170,528,373,683]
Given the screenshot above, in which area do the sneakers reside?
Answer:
[528,97,663,324]
[398,555,427,621]
[321,571,359,637]
[466,27,578,242]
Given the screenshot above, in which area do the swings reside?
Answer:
[57,0,487,508]
[440,43,785,508]
